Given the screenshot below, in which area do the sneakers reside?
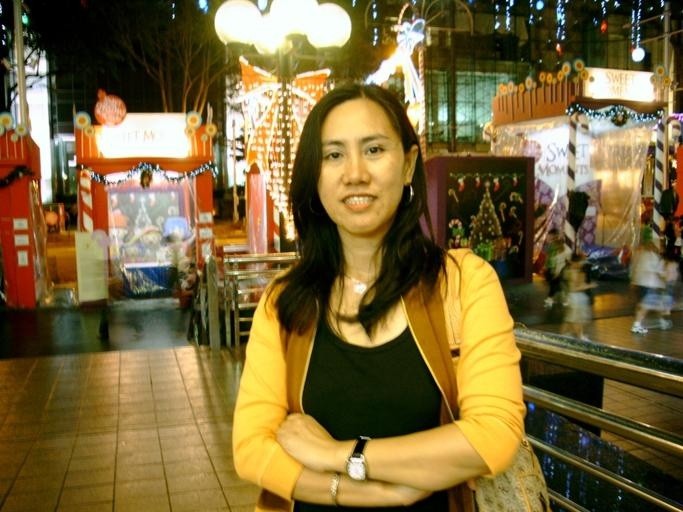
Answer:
[630,322,648,334]
[659,319,673,330]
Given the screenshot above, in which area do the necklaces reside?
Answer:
[344,271,381,294]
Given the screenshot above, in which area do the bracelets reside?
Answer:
[330,472,340,507]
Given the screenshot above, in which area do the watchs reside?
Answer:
[346,435,372,481]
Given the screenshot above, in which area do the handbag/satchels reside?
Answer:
[475,440,551,512]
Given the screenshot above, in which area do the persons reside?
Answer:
[543,200,680,343]
[232,84,550,512]
